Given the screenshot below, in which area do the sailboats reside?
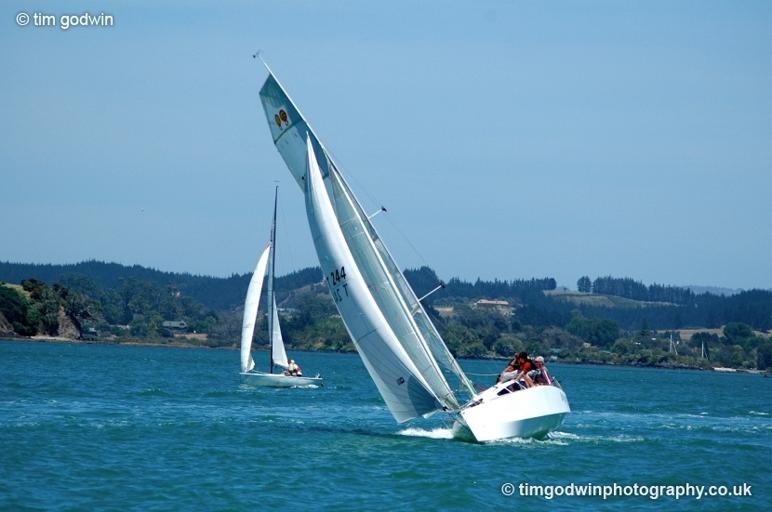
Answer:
[247,48,573,442]
[240,183,324,391]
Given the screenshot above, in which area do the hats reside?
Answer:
[514,351,545,363]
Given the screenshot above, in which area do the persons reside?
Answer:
[497,352,550,394]
[289,359,294,376]
